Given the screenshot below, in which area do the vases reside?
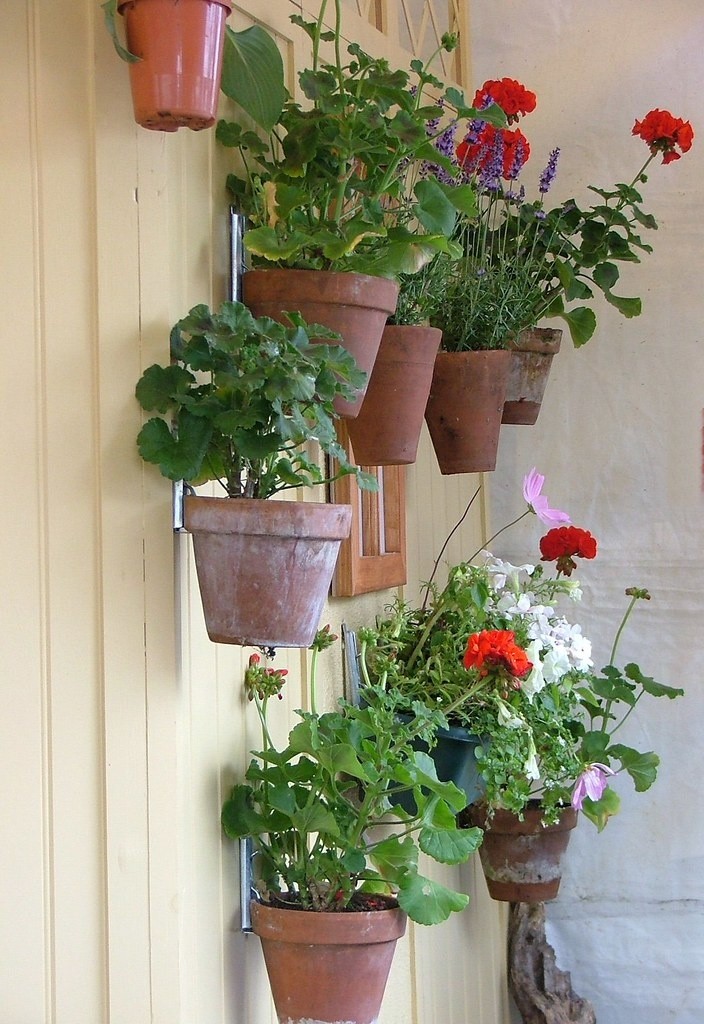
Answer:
[471,799,578,902]
[358,714,493,816]
[501,326,562,425]
[119,0,232,132]
[425,350,513,476]
[347,326,441,466]
[249,892,407,1024]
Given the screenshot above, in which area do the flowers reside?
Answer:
[377,77,695,354]
[218,469,686,925]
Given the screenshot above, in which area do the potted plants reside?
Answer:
[135,299,380,648]
[216,0,508,419]
